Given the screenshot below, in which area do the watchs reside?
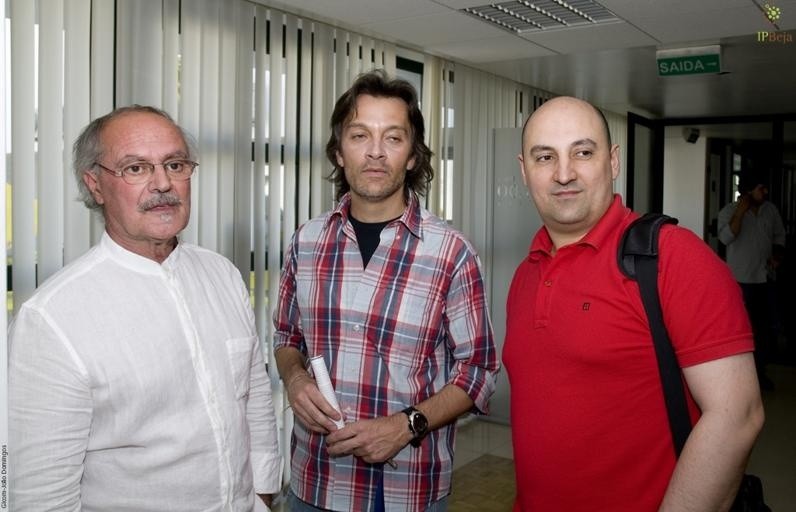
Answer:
[401,406,428,447]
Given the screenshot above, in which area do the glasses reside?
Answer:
[91,159,198,185]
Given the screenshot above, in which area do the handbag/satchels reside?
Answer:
[728,475,772,512]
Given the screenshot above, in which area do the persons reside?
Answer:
[8,105,282,512]
[502,96,767,512]
[718,178,786,388]
[274,69,501,512]
